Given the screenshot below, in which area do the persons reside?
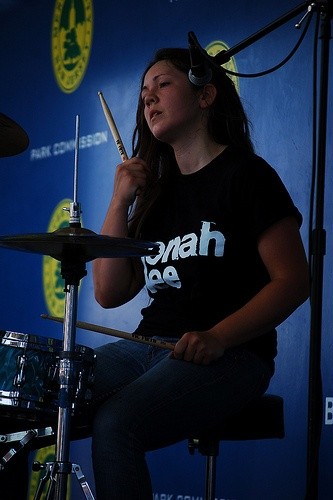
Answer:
[76,46,313,500]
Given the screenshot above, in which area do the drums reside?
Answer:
[0,329,97,419]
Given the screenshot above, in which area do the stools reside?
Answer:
[141,393,286,500]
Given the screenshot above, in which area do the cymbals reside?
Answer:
[1,227,159,263]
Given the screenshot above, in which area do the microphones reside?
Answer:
[188,31,213,88]
